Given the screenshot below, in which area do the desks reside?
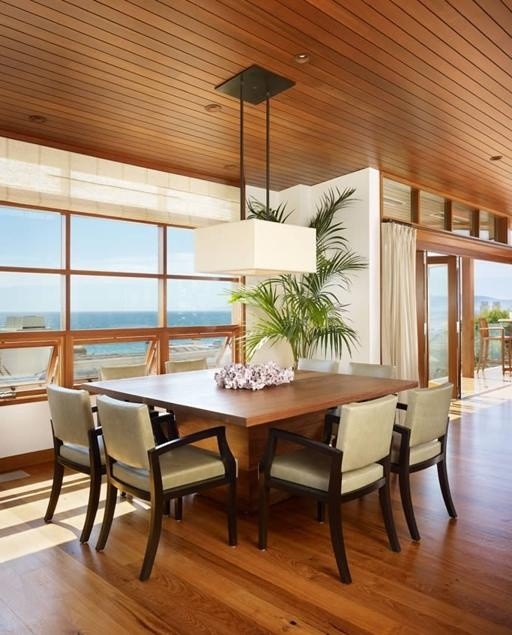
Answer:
[80,370,418,521]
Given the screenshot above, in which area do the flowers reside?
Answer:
[213,364,294,391]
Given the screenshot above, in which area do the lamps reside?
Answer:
[190,62,318,278]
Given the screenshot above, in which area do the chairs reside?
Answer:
[101,360,179,499]
[332,364,400,437]
[295,358,340,376]
[165,357,207,373]
[43,381,169,544]
[92,393,238,583]
[257,394,395,584]
[475,316,511,379]
[319,381,459,539]
